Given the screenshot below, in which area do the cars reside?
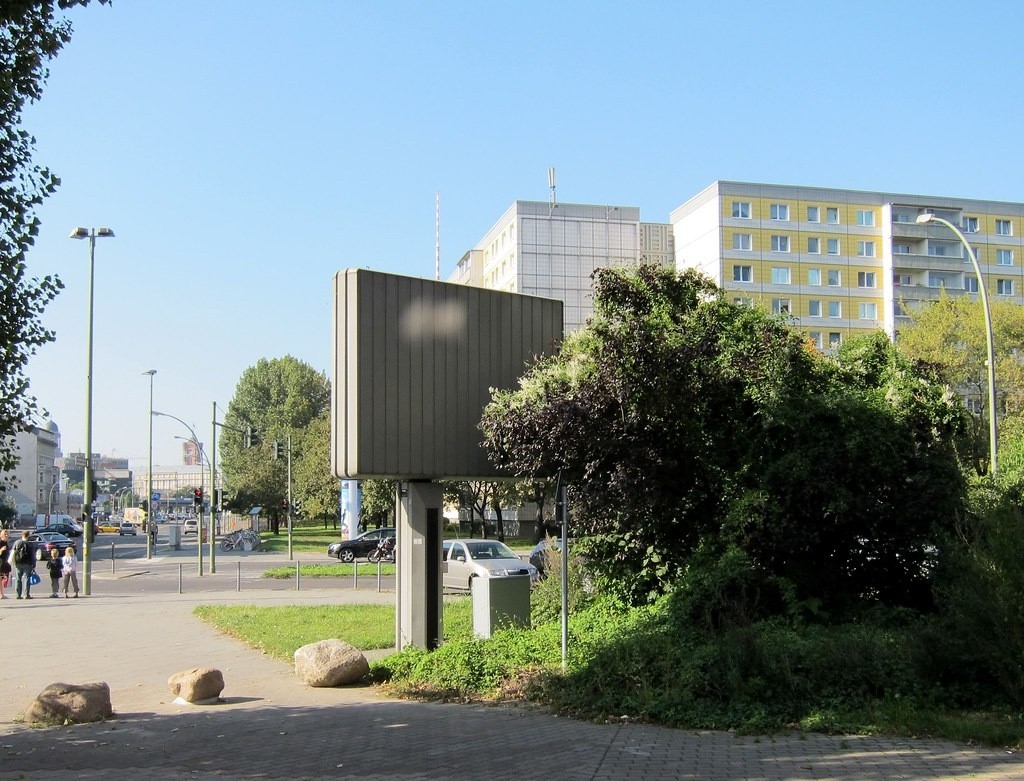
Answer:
[177,514,187,520]
[327,528,396,563]
[156,517,167,524]
[169,514,175,520]
[442,538,540,592]
[94,525,99,535]
[9,531,76,561]
[35,524,83,538]
[98,522,120,533]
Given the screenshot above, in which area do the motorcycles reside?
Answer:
[368,537,395,563]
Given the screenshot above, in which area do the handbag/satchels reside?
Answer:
[30,571,41,585]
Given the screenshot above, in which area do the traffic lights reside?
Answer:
[247,427,258,447]
[283,500,289,510]
[275,441,284,460]
[195,489,202,512]
[218,489,229,511]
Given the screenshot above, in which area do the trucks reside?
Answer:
[124,508,145,526]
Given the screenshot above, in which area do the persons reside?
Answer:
[0,529,11,598]
[47,547,79,598]
[12,530,36,599]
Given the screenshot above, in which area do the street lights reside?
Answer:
[95,484,142,515]
[48,477,70,515]
[142,370,158,559]
[191,456,228,490]
[916,212,997,478]
[69,226,115,595]
[151,410,203,575]
[173,435,210,481]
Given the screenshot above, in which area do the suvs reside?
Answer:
[120,521,137,535]
[528,538,572,580]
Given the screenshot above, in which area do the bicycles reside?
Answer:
[220,528,260,552]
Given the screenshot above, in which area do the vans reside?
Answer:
[184,520,198,535]
[36,514,77,531]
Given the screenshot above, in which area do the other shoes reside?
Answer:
[65,595,69,598]
[16,596,34,599]
[0,595,9,599]
[49,594,59,598]
[74,594,78,597]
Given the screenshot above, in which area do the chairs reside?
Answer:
[453,549,464,560]
[37,536,55,541]
[477,547,491,557]
